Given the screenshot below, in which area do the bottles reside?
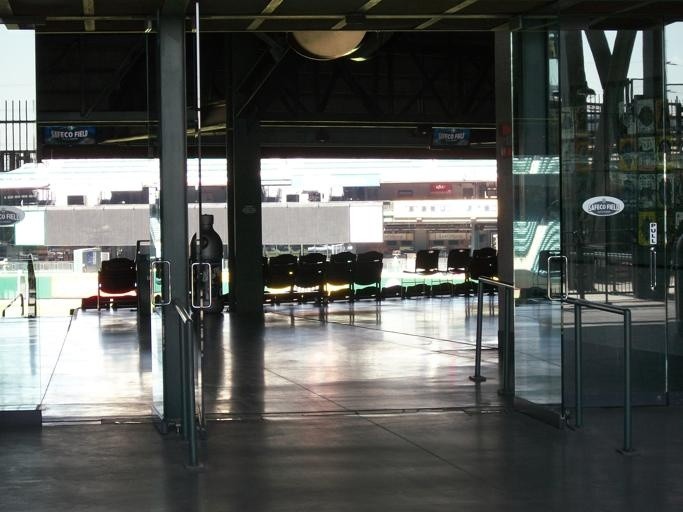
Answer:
[189,213,226,316]
